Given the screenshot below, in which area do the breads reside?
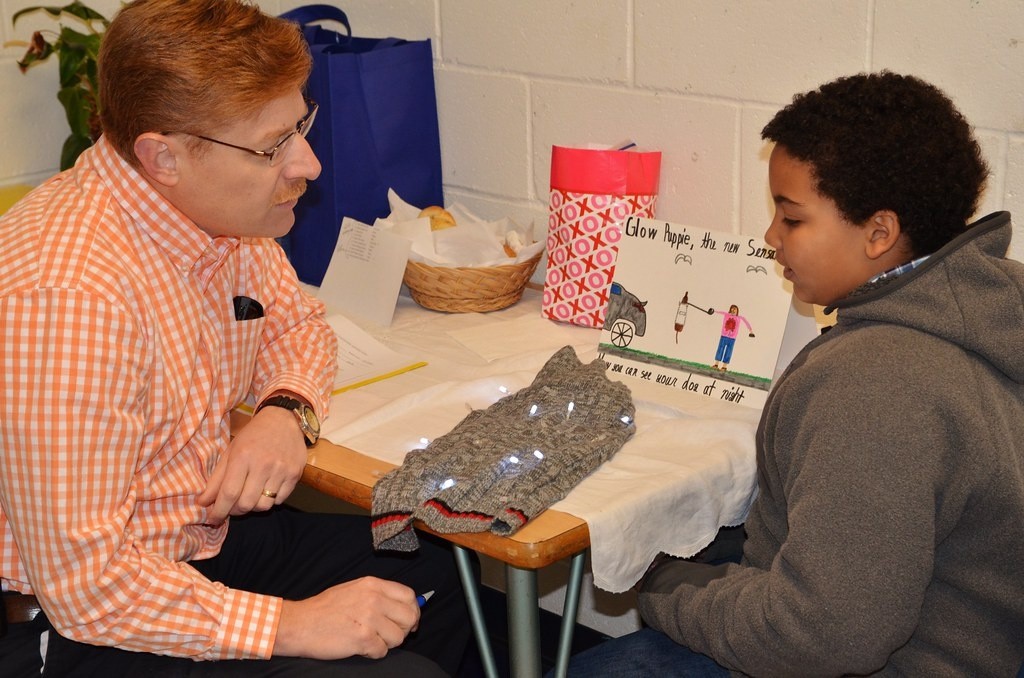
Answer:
[503,244,516,257]
[417,206,456,231]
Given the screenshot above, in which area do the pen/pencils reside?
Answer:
[418,590,435,607]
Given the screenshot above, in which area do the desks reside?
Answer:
[228,280,763,678]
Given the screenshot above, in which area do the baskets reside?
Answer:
[404,242,543,313]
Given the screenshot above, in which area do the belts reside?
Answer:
[3,595,41,625]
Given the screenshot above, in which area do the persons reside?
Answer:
[545,68,1024,678]
[0,0,482,678]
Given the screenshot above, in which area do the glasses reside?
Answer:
[159,94,319,167]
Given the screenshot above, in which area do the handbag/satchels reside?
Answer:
[542,141,662,329]
[277,3,444,287]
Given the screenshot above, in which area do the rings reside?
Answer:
[262,489,277,498]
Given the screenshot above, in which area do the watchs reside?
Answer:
[254,395,320,447]
